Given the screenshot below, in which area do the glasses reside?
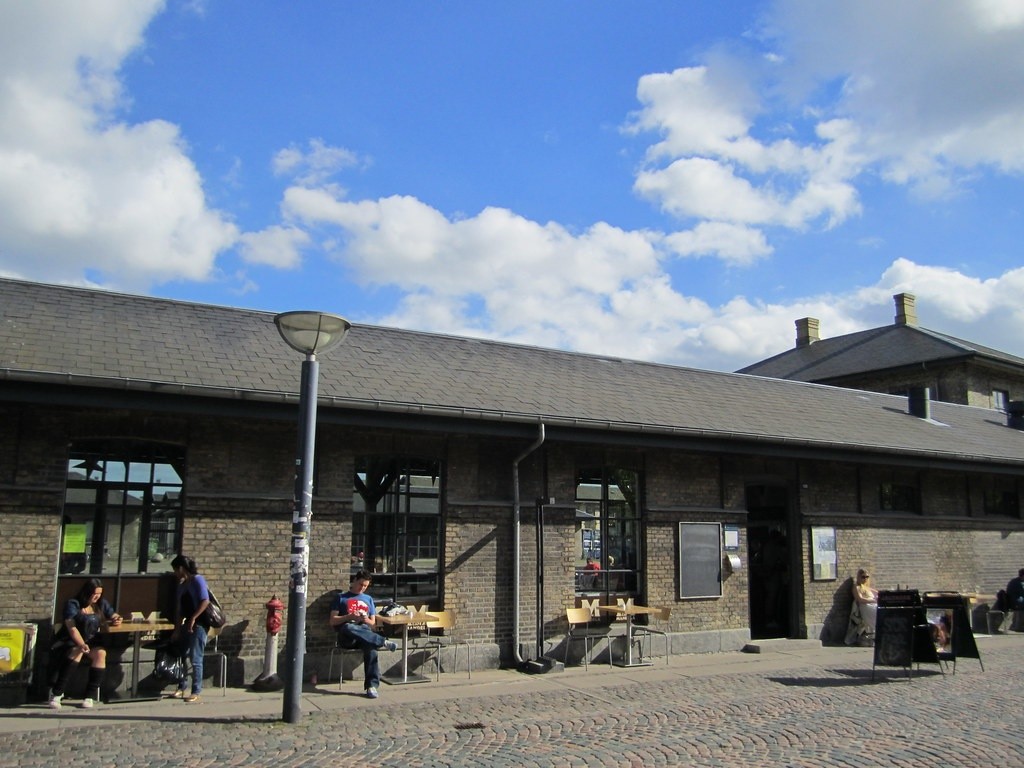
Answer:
[862,576,869,579]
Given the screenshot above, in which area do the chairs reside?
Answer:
[328,631,364,691]
[54,622,100,703]
[180,622,227,697]
[986,590,1024,636]
[420,611,471,682]
[631,605,671,665]
[563,608,613,672]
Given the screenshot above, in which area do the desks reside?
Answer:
[374,612,439,685]
[962,592,997,631]
[596,606,662,668]
[104,621,175,704]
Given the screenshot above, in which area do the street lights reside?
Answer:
[272,309,352,723]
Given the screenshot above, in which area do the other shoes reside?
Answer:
[384,640,397,652]
[170,689,183,697]
[82,697,94,708]
[49,687,64,709]
[367,687,378,698]
[186,694,199,702]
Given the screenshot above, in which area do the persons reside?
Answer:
[329,571,397,698]
[1006,568,1024,611]
[852,567,878,631]
[171,555,210,702]
[48,578,123,709]
[584,558,600,576]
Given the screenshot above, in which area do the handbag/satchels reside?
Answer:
[997,590,1010,612]
[203,585,226,628]
[153,641,183,682]
[850,611,862,626]
